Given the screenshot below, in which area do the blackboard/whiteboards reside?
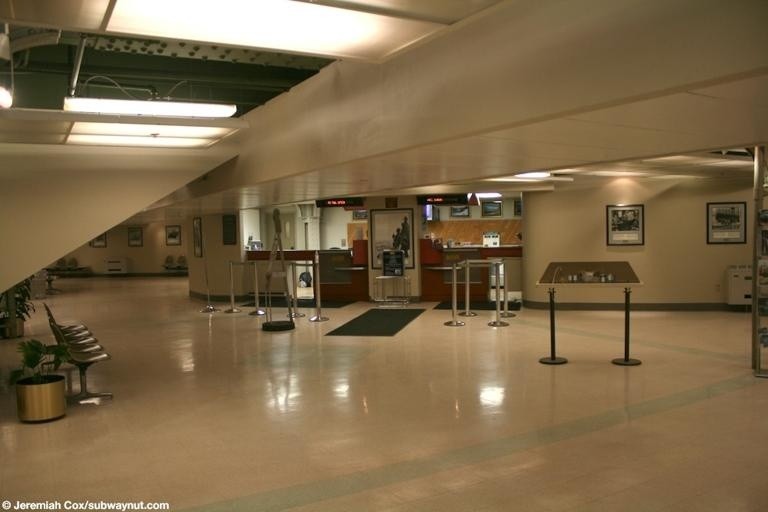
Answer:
[383,250,404,276]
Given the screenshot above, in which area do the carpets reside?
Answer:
[324,308,426,336]
[433,300,521,310]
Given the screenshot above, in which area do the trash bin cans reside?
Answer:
[726,264,753,305]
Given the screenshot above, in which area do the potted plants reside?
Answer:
[0,274,35,338]
[10,339,73,422]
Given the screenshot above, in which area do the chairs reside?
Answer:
[163,255,186,270]
[44,303,113,403]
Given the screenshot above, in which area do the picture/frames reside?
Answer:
[166,225,181,246]
[128,227,143,247]
[707,202,746,244]
[93,232,106,247]
[481,201,502,217]
[514,200,522,216]
[607,204,644,246]
[193,217,202,257]
[451,205,469,217]
